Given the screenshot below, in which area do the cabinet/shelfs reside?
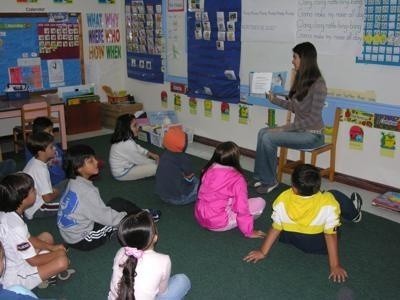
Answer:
[65,100,102,134]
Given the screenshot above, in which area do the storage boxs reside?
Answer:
[132,111,193,147]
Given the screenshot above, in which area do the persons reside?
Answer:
[251,42,327,195]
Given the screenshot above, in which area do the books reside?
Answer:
[248,71,274,98]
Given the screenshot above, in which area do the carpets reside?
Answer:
[1,129,399,298]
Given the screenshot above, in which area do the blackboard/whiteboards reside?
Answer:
[0,12,85,102]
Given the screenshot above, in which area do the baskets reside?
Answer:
[137,129,151,142]
[150,130,194,147]
[107,96,129,105]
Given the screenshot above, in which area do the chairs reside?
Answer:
[11,102,50,155]
[275,104,343,182]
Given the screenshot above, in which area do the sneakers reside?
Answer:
[255,181,279,194]
[350,191,363,223]
[250,179,263,188]
[38,274,57,290]
[142,208,162,222]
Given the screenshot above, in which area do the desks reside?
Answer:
[102,102,144,130]
[0,95,72,151]
